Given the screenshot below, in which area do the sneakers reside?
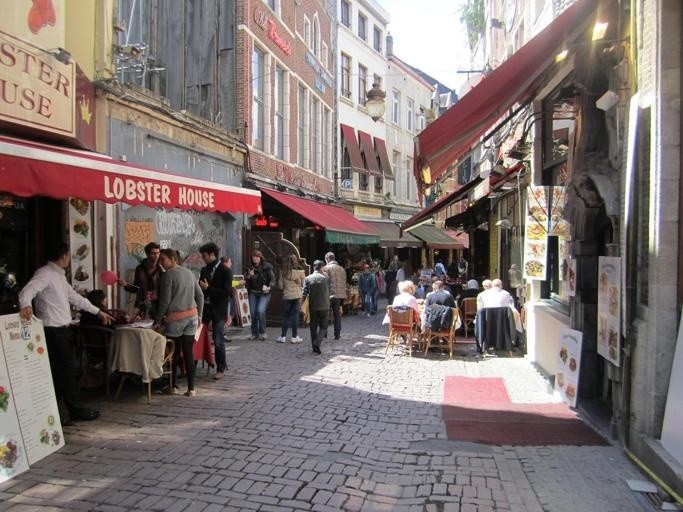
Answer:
[290,336,303,343]
[184,389,195,396]
[276,336,286,344]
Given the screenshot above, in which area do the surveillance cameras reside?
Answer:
[596,91,619,111]
[124,46,141,60]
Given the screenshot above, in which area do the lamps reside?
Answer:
[364,75,388,122]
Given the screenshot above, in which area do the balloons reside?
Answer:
[101,271,116,285]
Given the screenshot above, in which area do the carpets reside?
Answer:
[441,374,616,448]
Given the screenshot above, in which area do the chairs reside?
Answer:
[70,308,173,405]
[381,277,524,361]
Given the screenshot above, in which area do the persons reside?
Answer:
[435,255,468,279]
[152,249,204,398]
[198,242,234,378]
[117,242,165,318]
[393,276,514,354]
[17,239,116,422]
[78,289,121,360]
[243,251,347,355]
[220,256,233,342]
[344,255,433,316]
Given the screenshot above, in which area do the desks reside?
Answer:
[116,318,216,377]
